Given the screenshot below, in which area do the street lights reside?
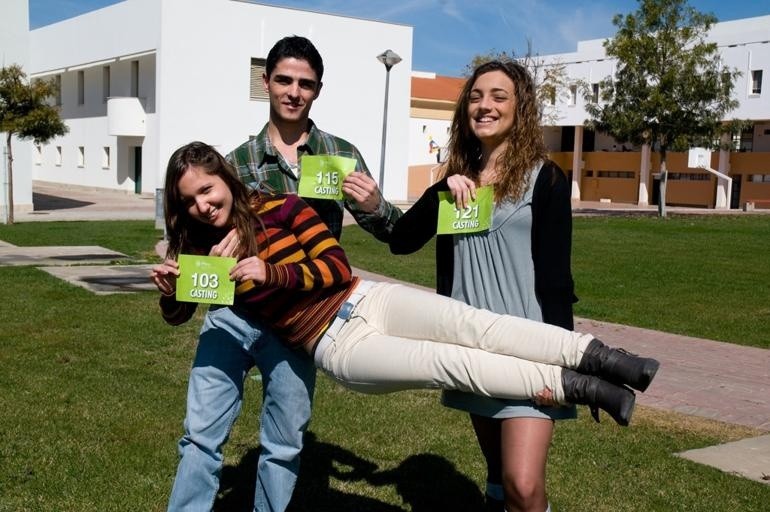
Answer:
[376,49,402,194]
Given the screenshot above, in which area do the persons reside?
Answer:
[148,141,659,428]
[166,35,403,511]
[390,61,579,511]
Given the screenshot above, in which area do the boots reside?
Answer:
[561,367,635,426]
[577,337,661,392]
